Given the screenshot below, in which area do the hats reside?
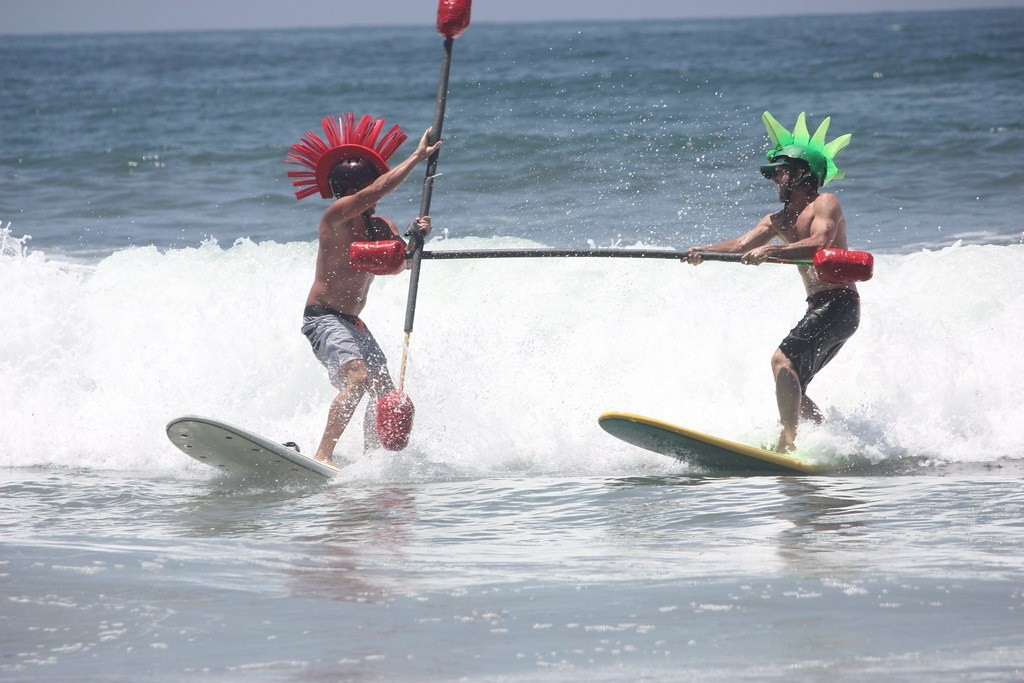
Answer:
[284,112,408,201]
[760,110,852,187]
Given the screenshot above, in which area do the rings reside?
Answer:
[744,261,747,264]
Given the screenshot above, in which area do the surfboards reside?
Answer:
[166,415,344,480]
[594,410,861,475]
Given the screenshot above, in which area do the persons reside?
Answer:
[681,145,860,453]
[301,126,443,464]
